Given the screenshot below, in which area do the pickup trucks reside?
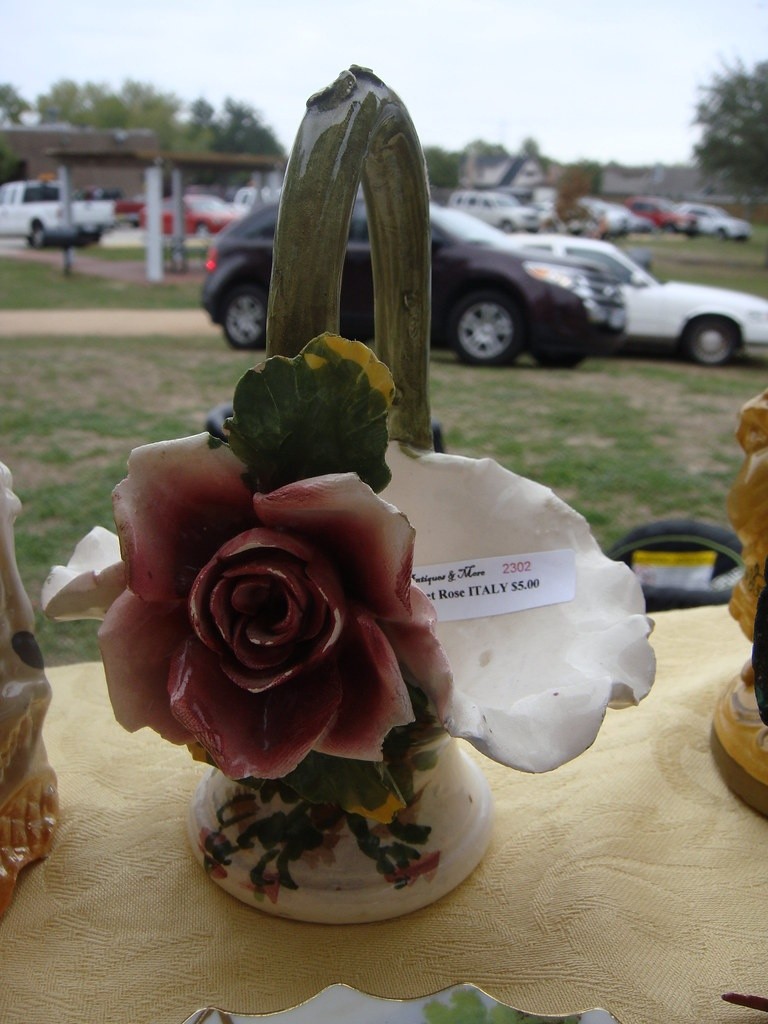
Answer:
[1,180,119,251]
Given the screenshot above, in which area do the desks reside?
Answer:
[2,601,768,1024]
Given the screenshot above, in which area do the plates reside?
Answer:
[178,982,622,1024]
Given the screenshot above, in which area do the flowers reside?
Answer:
[35,332,657,829]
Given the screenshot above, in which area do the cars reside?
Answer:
[139,192,251,236]
[202,197,628,371]
[505,225,767,367]
[447,190,540,234]
[515,180,751,242]
